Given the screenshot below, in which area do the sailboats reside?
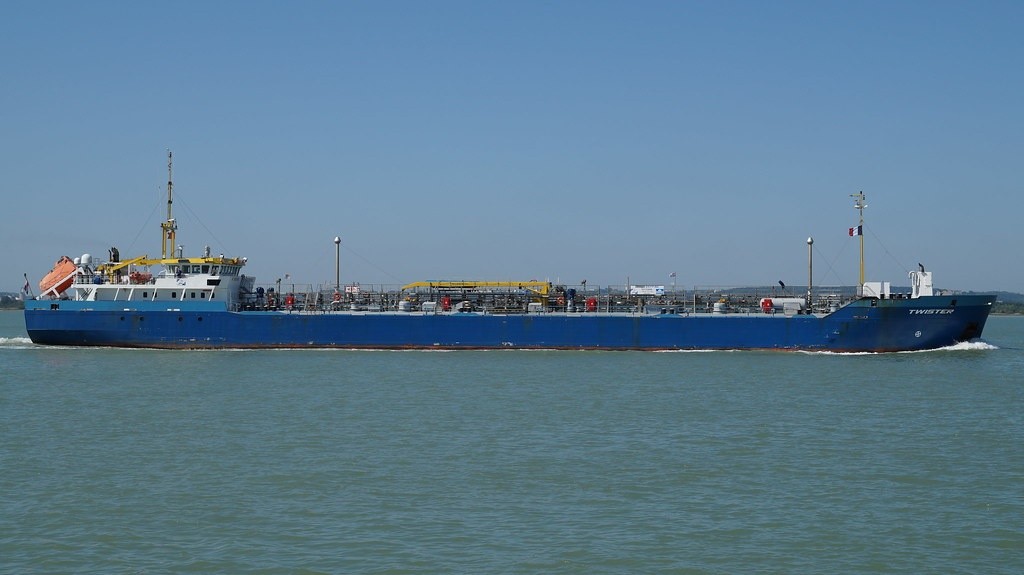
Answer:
[22,150,1000,353]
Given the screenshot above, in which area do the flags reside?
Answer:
[848,225,862,237]
[24,281,30,295]
